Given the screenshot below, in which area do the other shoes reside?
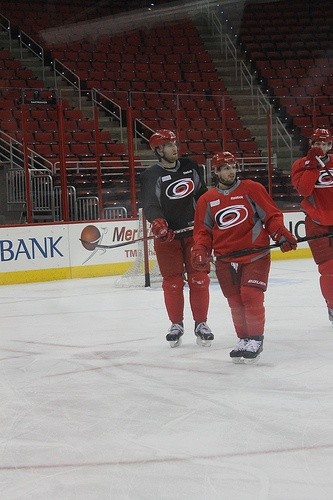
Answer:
[328,308,333,321]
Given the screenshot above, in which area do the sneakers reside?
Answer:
[193,321,214,347]
[229,336,248,364]
[165,321,184,348]
[242,335,264,365]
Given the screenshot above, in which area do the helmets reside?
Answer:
[149,129,177,153]
[309,128,333,147]
[211,151,238,169]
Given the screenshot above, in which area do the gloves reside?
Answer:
[271,225,298,253]
[149,217,176,244]
[305,147,324,168]
[189,245,211,274]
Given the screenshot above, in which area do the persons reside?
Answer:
[140,128,215,342]
[190,151,298,358]
[290,129,333,322]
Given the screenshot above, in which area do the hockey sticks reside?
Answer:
[205,232,333,263]
[314,155,333,182]
[78,225,195,249]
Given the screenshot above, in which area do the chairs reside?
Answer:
[0,0,333,220]
